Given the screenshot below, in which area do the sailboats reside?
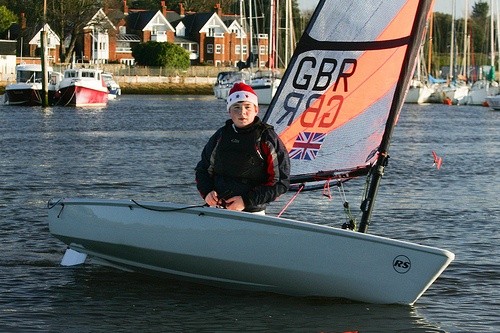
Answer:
[212,0,500,111]
[45,0,456,306]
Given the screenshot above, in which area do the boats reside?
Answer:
[102,72,122,101]
[56,51,109,109]
[5,61,62,103]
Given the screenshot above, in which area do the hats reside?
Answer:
[227,82,259,113]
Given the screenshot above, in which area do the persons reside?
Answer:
[195,83,290,216]
